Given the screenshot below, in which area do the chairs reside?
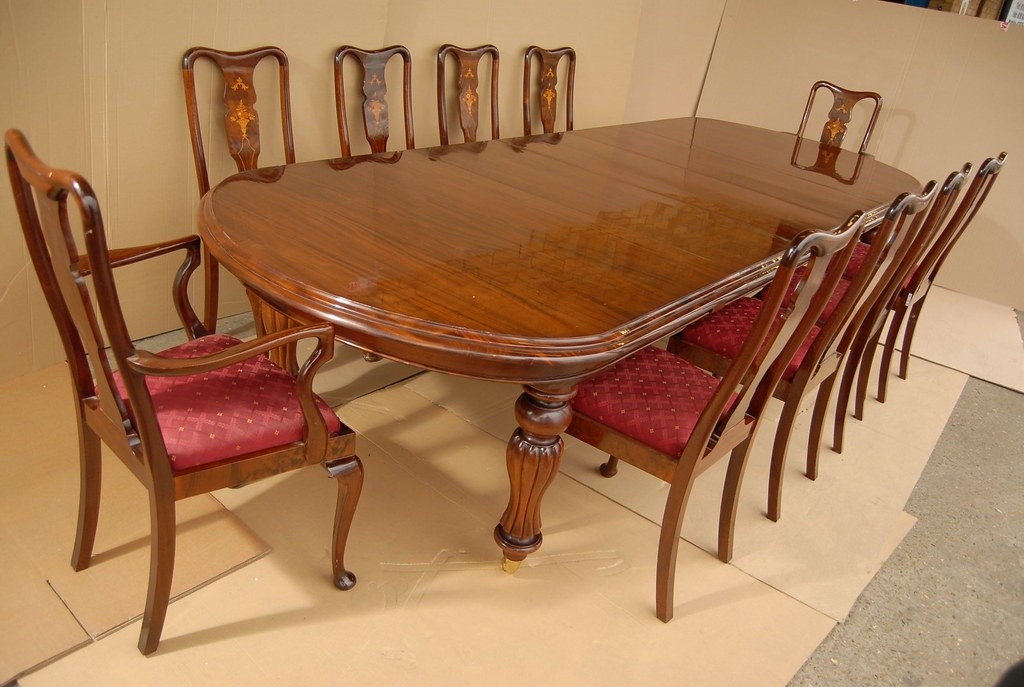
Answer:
[4,128,367,658]
[521,46,577,136]
[181,46,305,334]
[798,82,884,151]
[756,160,972,421]
[552,206,866,622]
[836,149,1009,403]
[436,44,502,150]
[334,44,420,157]
[663,178,941,570]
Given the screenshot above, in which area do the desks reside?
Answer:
[198,114,924,577]
